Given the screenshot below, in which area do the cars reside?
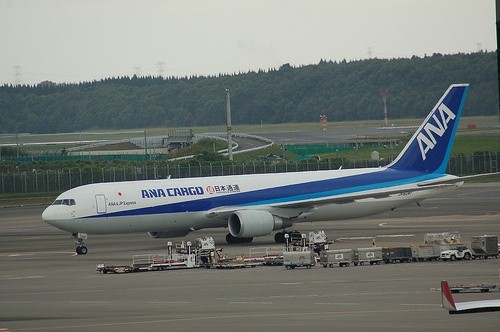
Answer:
[439,246,472,261]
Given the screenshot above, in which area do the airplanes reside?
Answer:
[42,83,500,255]
